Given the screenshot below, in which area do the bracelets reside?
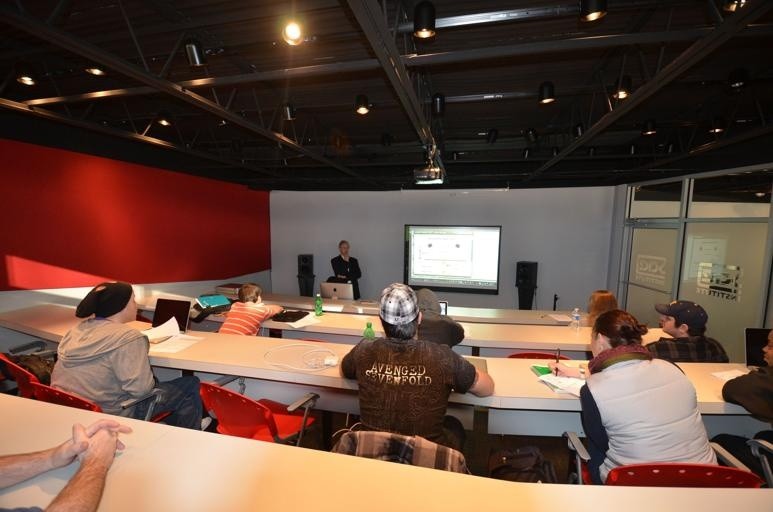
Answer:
[579,364,587,379]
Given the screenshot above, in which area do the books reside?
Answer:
[215,284,245,300]
[531,364,571,394]
[195,295,231,309]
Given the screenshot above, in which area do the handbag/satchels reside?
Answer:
[5,352,53,386]
[189,294,233,323]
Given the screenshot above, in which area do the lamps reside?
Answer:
[577,0,607,22]
[572,124,585,140]
[354,94,370,115]
[642,120,657,135]
[708,116,726,133]
[412,1,436,39]
[525,127,538,144]
[538,80,556,104]
[486,130,499,144]
[611,75,632,99]
[431,93,445,117]
[185,40,224,66]
[282,23,317,46]
[282,102,297,121]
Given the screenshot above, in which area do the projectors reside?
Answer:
[414,167,444,185]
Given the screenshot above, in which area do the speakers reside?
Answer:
[516,261,538,286]
[297,254,314,276]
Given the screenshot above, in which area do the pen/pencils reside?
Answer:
[556,348,560,377]
[541,314,546,318]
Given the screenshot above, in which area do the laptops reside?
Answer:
[320,282,354,300]
[146,298,192,344]
[438,302,447,315]
[743,326,773,367]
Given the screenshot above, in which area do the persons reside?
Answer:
[709,327,773,489]
[50,281,201,431]
[571,291,620,326]
[644,300,731,363]
[218,282,284,337]
[415,286,464,349]
[0,418,133,512]
[330,240,361,303]
[569,309,720,486]
[339,283,495,452]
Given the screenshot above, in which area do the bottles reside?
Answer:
[571,308,581,334]
[363,322,375,338]
[315,294,322,316]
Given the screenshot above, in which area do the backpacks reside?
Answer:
[488,445,567,484]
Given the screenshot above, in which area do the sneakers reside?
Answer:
[200,416,212,431]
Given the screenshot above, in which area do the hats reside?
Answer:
[654,300,708,329]
[379,282,420,326]
[76,282,132,318]
